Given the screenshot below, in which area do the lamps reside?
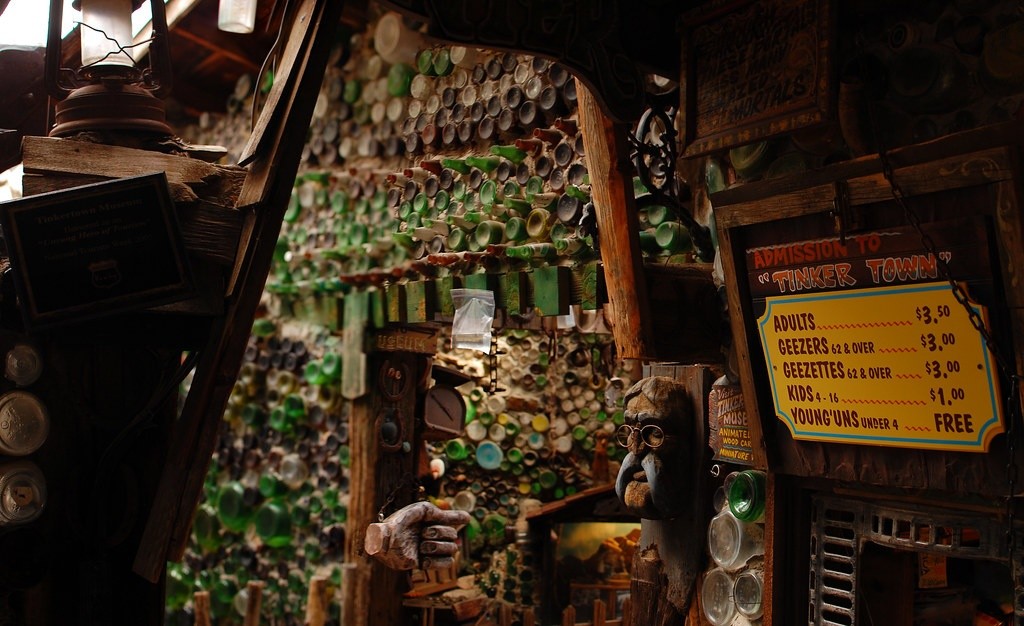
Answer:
[47,0,178,147]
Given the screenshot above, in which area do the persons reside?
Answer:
[614,375,687,511]
[364,502,471,571]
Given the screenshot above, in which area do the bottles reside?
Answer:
[174,13,768,626]
